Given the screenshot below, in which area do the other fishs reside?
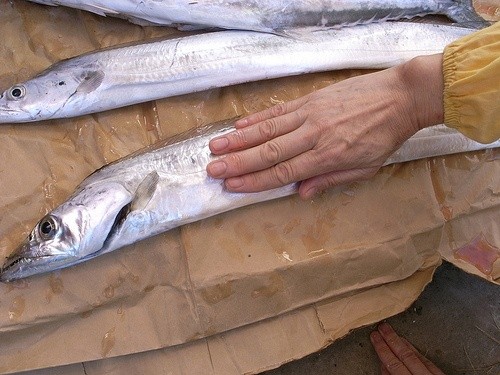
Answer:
[0,107,500,285]
[0,0,493,124]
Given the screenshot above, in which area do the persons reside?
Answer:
[207,20,499,375]
